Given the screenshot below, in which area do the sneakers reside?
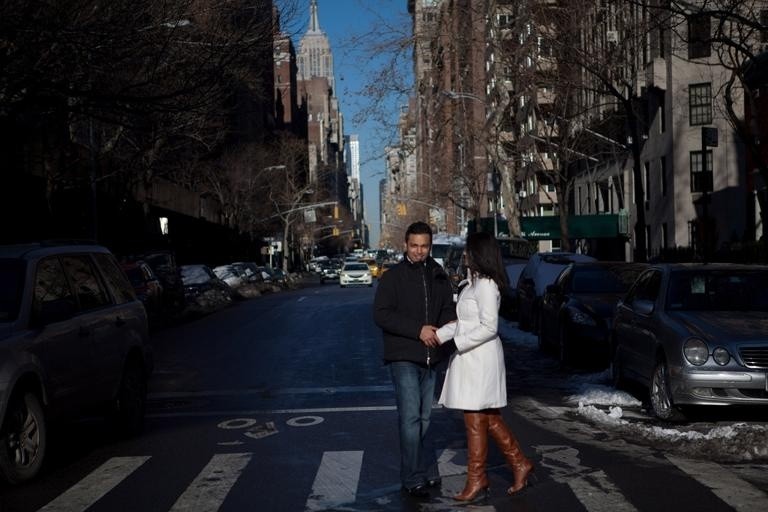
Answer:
[402,478,441,503]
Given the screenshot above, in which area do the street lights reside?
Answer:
[406,171,439,211]
[245,164,290,197]
[293,214,355,241]
[435,86,500,236]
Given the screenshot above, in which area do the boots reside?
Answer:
[450,411,490,503]
[488,413,539,496]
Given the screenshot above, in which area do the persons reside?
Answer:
[434,231,535,502]
[372,223,457,495]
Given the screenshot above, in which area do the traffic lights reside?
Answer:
[430,215,436,224]
[396,204,403,216]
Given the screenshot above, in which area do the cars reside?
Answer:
[307,248,404,288]
